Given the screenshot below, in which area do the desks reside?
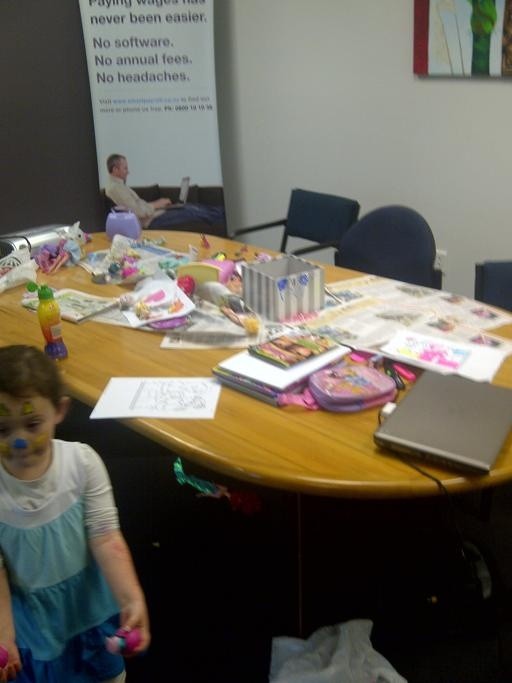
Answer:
[0,227,512,645]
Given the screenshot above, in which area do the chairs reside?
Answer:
[227,188,512,312]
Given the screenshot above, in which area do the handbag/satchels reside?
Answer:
[309,365,399,414]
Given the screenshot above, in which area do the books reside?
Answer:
[212,326,351,410]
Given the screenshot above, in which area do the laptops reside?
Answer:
[372,370,512,476]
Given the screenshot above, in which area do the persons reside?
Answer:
[105,154,172,229]
[0,343,151,682]
[35,221,92,274]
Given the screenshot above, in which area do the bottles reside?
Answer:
[29,281,69,359]
